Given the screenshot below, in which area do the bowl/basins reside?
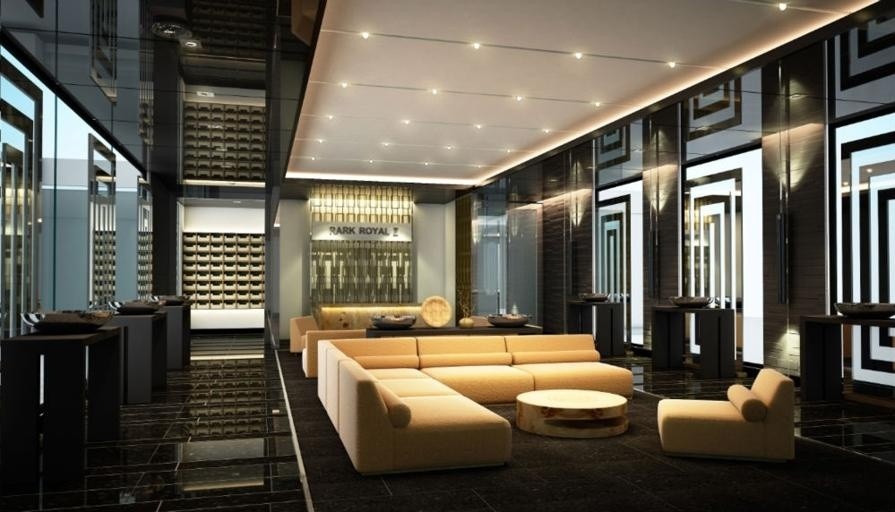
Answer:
[487,314,529,327]
[369,314,417,328]
[20,306,112,335]
[578,292,609,302]
[108,294,192,313]
[833,303,895,318]
[668,295,713,308]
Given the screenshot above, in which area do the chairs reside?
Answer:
[657,367,795,463]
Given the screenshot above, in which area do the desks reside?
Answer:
[0,304,191,457]
[567,301,625,358]
[800,315,895,401]
[652,305,734,379]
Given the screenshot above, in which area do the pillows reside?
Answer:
[727,384,767,422]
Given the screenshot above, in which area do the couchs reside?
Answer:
[289,304,423,379]
[316,333,633,476]
